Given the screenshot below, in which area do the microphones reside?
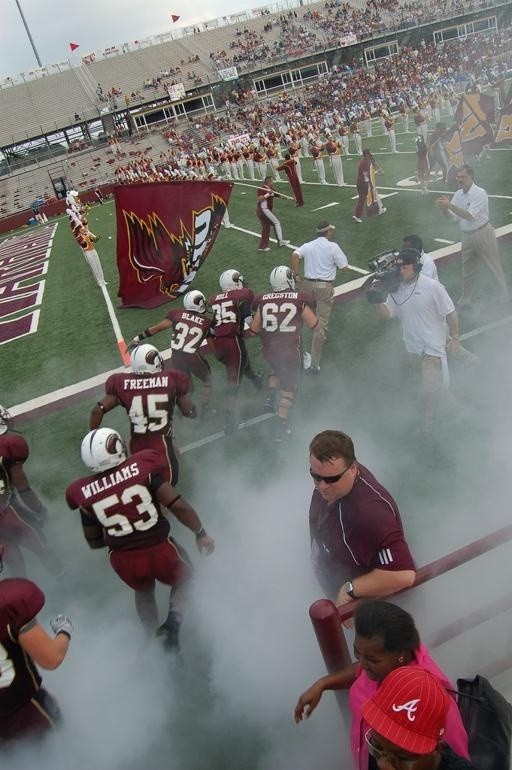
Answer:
[407,265,423,280]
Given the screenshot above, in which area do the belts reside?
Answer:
[461,220,491,235]
[304,277,334,283]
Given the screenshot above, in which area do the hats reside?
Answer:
[73,224,82,234]
[70,219,75,227]
[159,91,461,182]
[396,248,421,264]
[359,665,450,755]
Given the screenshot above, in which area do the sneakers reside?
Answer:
[377,207,387,216]
[223,419,247,435]
[352,215,364,223]
[224,223,235,229]
[421,185,427,196]
[264,397,277,414]
[304,366,321,380]
[258,246,272,252]
[421,412,435,440]
[199,409,217,424]
[253,368,265,388]
[338,182,348,187]
[156,618,183,664]
[278,239,291,247]
[303,351,312,370]
[274,427,294,442]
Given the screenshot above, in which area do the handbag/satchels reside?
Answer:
[437,673,512,770]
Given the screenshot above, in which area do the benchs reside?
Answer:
[0,1,510,217]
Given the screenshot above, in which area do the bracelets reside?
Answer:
[193,528,208,538]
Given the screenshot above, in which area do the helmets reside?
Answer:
[218,269,246,292]
[129,343,164,376]
[0,405,12,436]
[78,426,127,473]
[182,289,206,314]
[270,265,296,293]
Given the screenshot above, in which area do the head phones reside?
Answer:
[404,247,423,274]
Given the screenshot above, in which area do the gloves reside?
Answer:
[125,335,140,355]
[49,614,75,640]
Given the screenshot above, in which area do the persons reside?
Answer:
[90,345,196,490]
[308,430,417,630]
[63,428,217,655]
[0,405,64,587]
[358,666,470,770]
[293,600,471,770]
[0,579,74,752]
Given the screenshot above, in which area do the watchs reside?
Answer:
[344,577,356,600]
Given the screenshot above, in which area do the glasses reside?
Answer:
[309,464,351,485]
[364,728,421,770]
